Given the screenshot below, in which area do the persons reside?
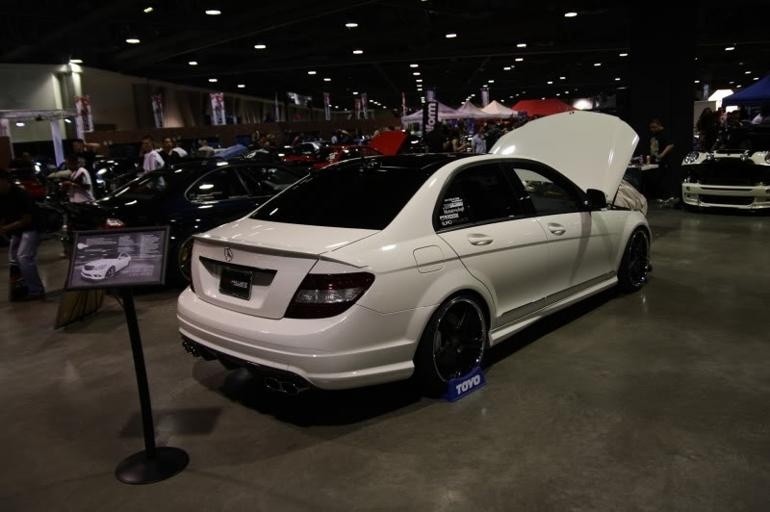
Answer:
[399,115,541,154]
[0,166,48,299]
[648,118,682,209]
[214,94,225,124]
[50,132,212,206]
[79,96,92,131]
[153,95,164,128]
[692,105,770,151]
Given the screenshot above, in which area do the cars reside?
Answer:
[1,124,690,285]
[678,124,770,217]
[183,110,656,397]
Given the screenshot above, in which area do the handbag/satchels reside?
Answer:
[8,260,29,301]
[36,202,63,233]
